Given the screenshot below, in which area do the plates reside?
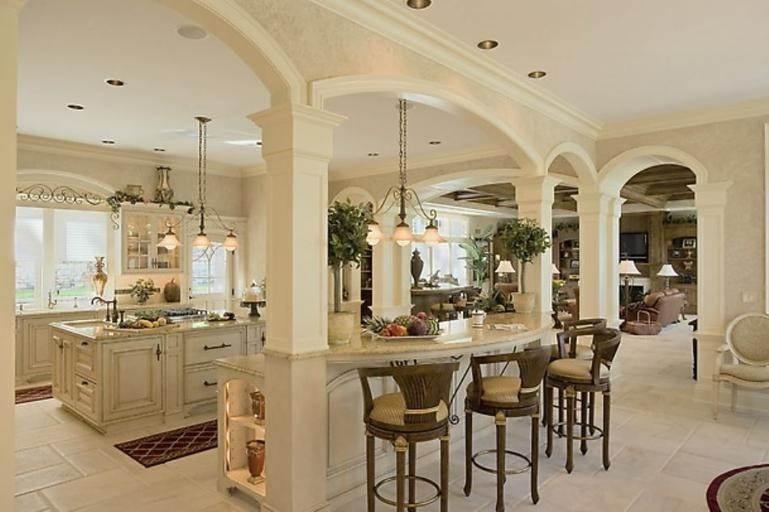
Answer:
[111,324,174,332]
[367,329,440,340]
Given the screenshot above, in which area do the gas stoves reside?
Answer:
[164,306,208,319]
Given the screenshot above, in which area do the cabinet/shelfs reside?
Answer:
[410,279,475,321]
[666,236,697,314]
[107,202,184,305]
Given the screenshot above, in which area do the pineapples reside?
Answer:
[361,314,415,337]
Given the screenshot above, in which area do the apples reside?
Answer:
[407,319,426,336]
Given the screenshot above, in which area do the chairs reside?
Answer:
[712,312,769,421]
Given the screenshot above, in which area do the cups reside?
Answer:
[473,310,485,327]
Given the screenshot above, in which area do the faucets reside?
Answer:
[47,290,57,308]
[90,297,119,324]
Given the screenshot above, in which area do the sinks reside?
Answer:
[63,319,114,329]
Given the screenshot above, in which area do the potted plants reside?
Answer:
[328,196,381,342]
[498,217,552,313]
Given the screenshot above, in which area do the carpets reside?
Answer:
[706,463,769,512]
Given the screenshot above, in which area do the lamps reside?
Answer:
[495,260,517,283]
[363,98,448,248]
[552,263,561,274]
[656,263,679,289]
[618,260,642,332]
[155,116,240,251]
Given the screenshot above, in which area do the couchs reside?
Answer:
[620,285,684,335]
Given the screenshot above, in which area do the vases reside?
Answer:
[125,184,146,200]
[91,254,107,300]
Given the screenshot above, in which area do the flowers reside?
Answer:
[128,278,154,300]
